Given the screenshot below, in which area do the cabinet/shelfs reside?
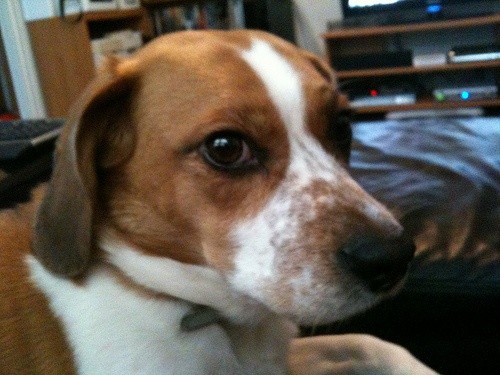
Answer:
[319,14,500,125]
[24,8,157,120]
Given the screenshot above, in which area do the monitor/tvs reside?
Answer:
[340,0,500,21]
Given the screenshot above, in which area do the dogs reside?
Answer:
[0,27,420,374]
